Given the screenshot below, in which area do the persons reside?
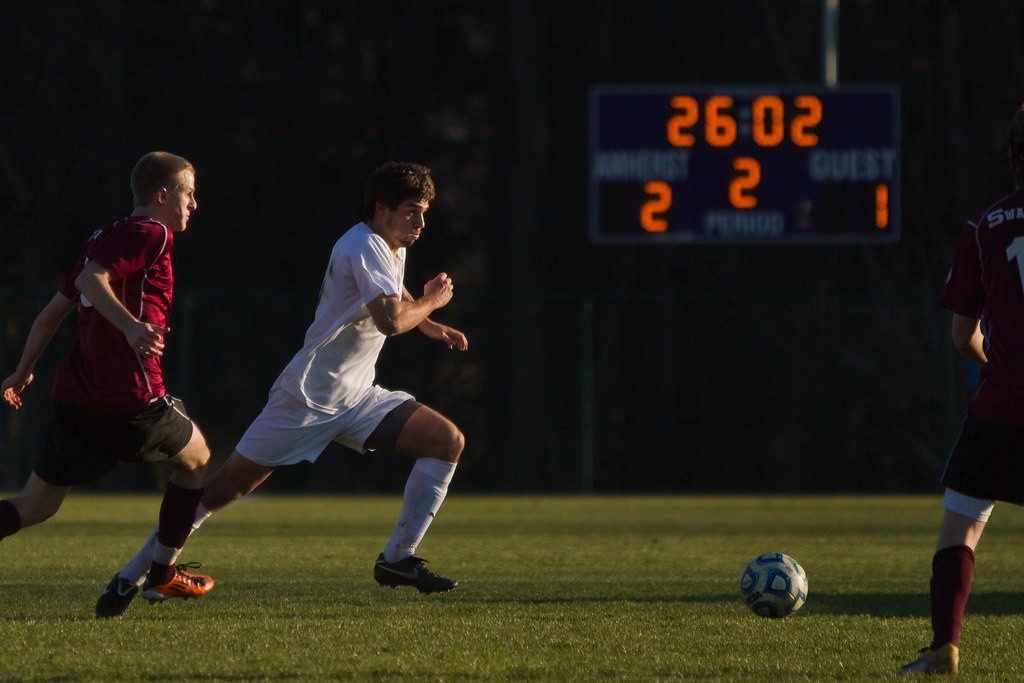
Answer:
[899,110,1024,672]
[0,150,215,605]
[94,161,468,618]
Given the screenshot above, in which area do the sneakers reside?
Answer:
[900,642,960,677]
[374,553,458,594]
[96,571,139,619]
[141,562,215,605]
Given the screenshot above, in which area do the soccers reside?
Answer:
[739,551,810,619]
